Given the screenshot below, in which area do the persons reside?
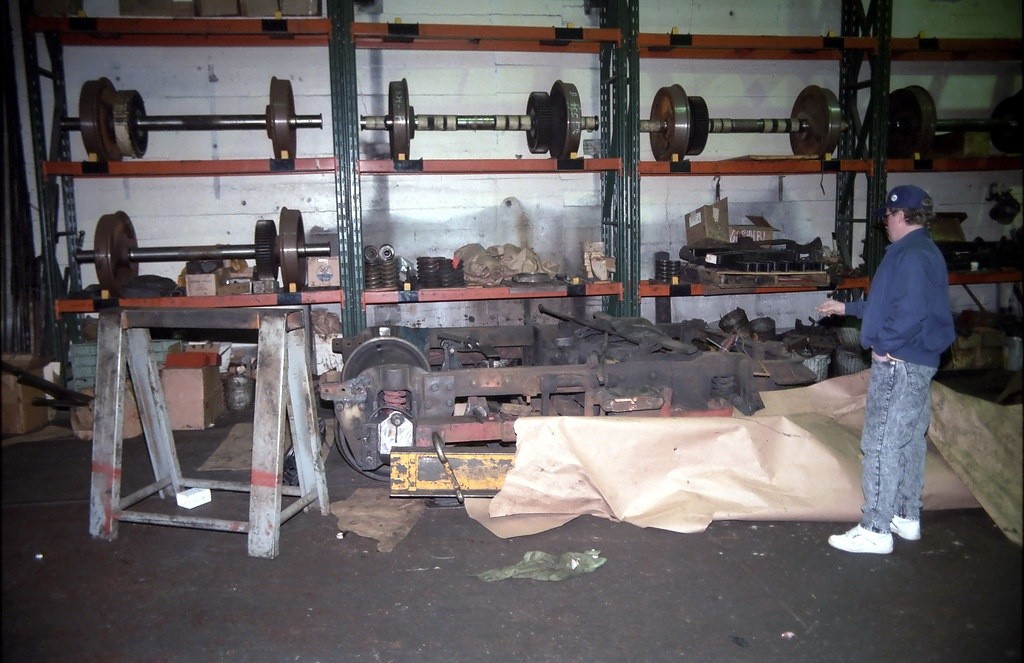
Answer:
[812,182,956,557]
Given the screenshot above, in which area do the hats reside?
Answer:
[871,183,932,216]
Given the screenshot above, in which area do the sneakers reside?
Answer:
[828,524,893,554]
[889,514,922,541]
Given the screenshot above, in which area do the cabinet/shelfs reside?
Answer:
[17,0,1024,437]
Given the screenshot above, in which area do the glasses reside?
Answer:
[881,210,901,221]
